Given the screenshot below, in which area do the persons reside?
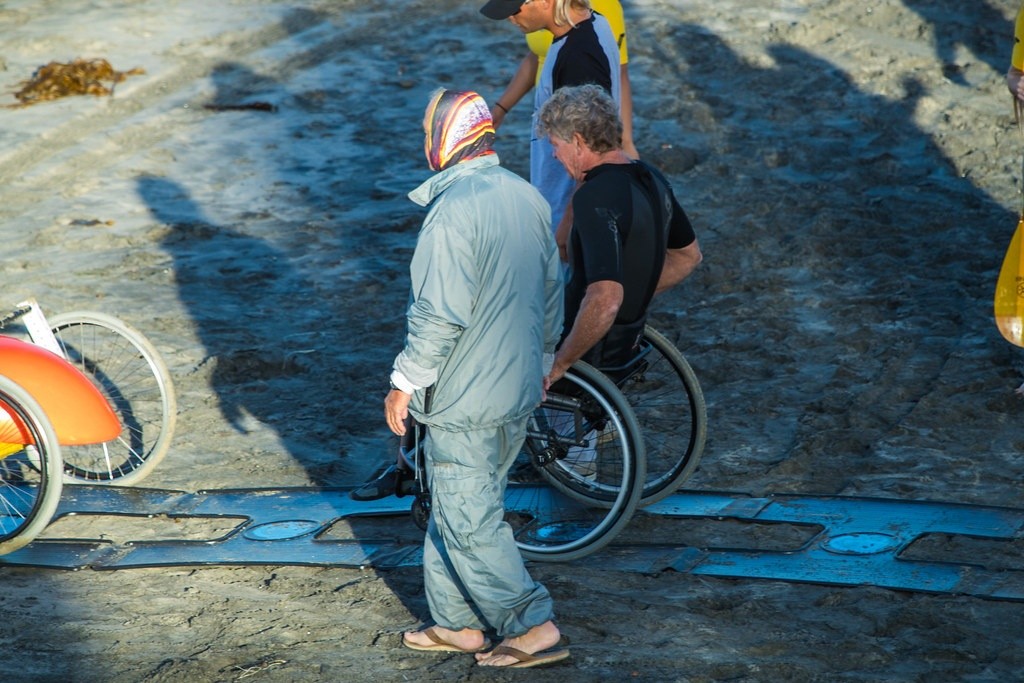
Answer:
[484,0,639,486]
[1006,3,1024,128]
[549,85,701,404]
[380,87,569,670]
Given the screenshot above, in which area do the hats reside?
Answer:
[478,1,527,21]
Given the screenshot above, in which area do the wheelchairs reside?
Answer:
[0,296,178,558]
[349,320,710,563]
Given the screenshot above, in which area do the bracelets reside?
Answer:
[390,381,402,392]
[496,102,508,113]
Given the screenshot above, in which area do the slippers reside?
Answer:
[475,644,571,671]
[403,623,491,654]
